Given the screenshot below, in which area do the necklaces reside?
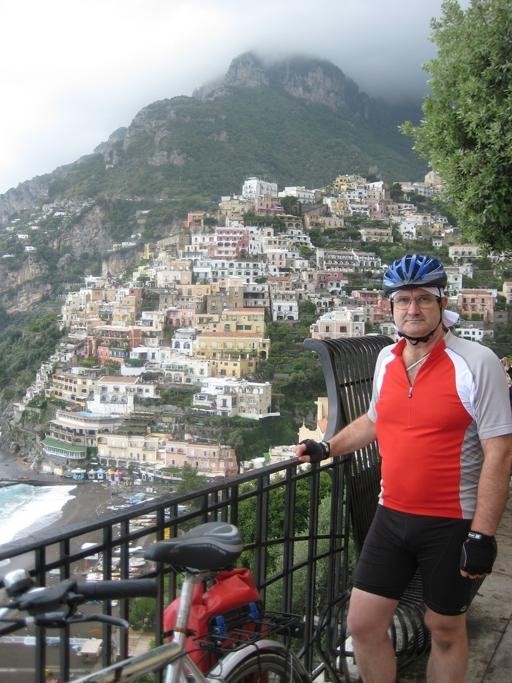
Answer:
[405,351,432,371]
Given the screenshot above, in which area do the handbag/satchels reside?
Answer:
[164,569,268,683]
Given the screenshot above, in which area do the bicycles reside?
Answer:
[0,519,314,683]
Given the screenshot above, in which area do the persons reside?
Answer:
[289,252,512,682]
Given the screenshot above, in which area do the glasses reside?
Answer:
[392,297,439,309]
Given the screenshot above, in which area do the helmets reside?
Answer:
[382,253,447,297]
